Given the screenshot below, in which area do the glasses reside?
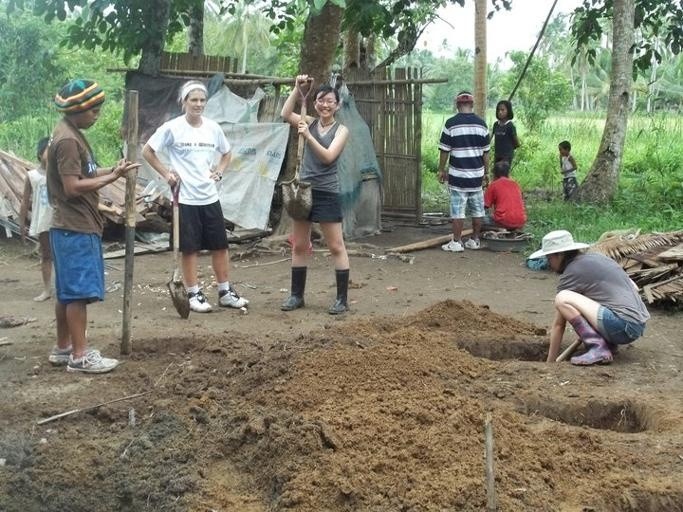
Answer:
[316,98,337,106]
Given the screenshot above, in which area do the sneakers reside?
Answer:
[463,236,480,250]
[441,238,465,252]
[66,350,118,374]
[188,288,213,313]
[48,344,100,363]
[218,286,250,308]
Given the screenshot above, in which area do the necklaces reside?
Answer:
[318,116,335,127]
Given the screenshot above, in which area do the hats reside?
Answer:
[528,230,590,260]
[55,80,105,113]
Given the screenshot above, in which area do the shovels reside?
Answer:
[280,78,312,220]
[165,178,190,320]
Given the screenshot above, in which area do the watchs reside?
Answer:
[215,170,223,179]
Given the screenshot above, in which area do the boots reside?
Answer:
[328,269,350,314]
[280,267,307,311]
[568,313,612,365]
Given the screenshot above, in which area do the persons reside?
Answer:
[558,141,577,201]
[20,137,54,303]
[46,82,142,373]
[280,74,351,315]
[478,161,527,236]
[491,99,519,178]
[528,229,650,366]
[437,91,490,252]
[142,80,249,313]
